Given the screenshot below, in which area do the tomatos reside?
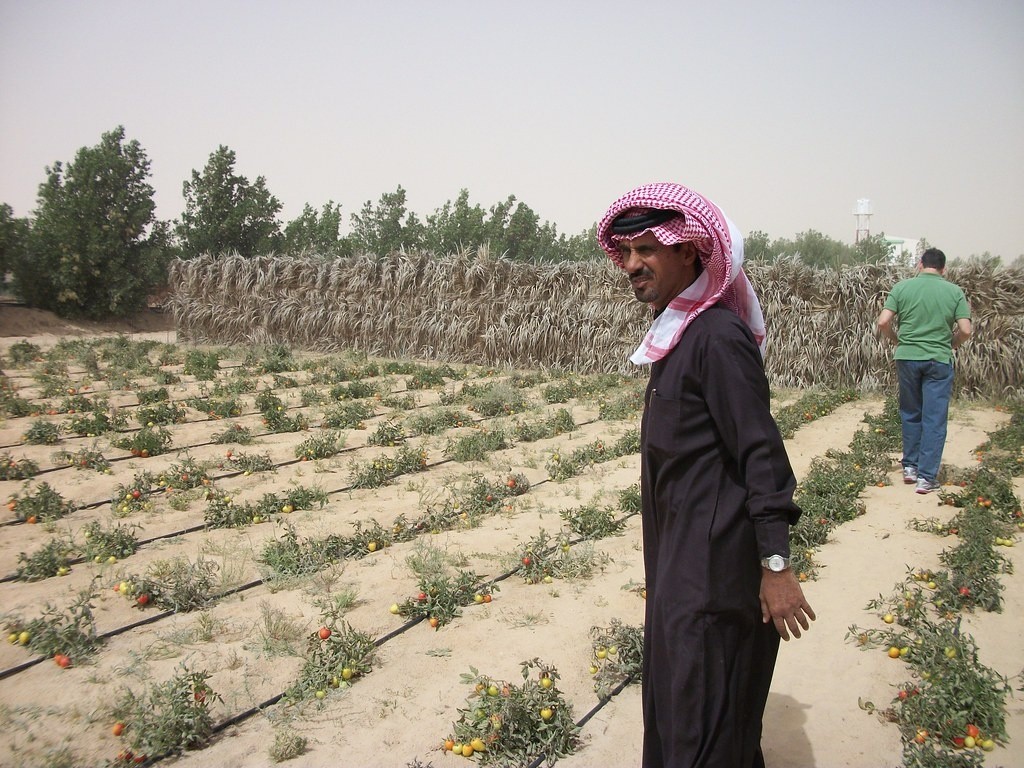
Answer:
[0,347,1021,764]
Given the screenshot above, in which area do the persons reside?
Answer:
[595,182,815,768]
[879,248,972,494]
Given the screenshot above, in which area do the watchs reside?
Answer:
[761,554,790,572]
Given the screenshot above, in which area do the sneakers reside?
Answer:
[914,478,941,494]
[902,466,917,484]
